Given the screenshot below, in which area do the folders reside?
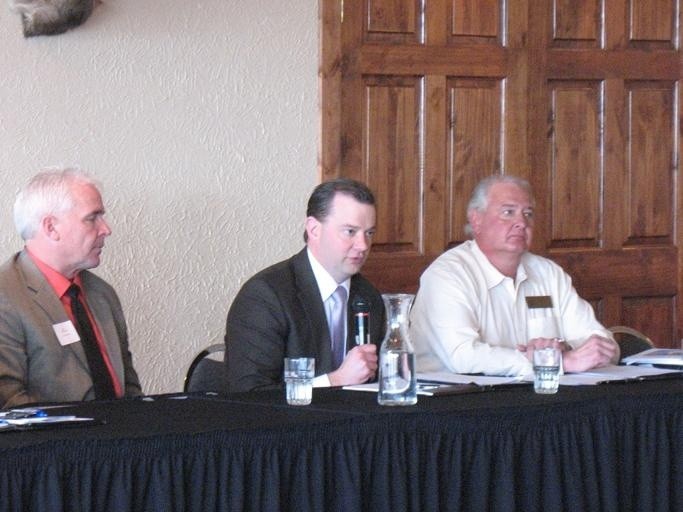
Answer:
[341,371,533,397]
[559,365,683,387]
[0,409,96,433]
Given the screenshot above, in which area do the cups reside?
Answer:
[283,359,314,405]
[534,349,564,393]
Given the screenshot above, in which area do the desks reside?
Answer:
[0,366,683,510]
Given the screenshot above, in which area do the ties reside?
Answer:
[64,283,116,403]
[325,286,351,372]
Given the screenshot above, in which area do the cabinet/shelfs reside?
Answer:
[530,0,683,351]
[320,0,528,293]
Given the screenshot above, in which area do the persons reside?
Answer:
[407,175,622,377]
[0,168,144,409]
[223,179,388,394]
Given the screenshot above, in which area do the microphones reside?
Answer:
[350,294,371,352]
